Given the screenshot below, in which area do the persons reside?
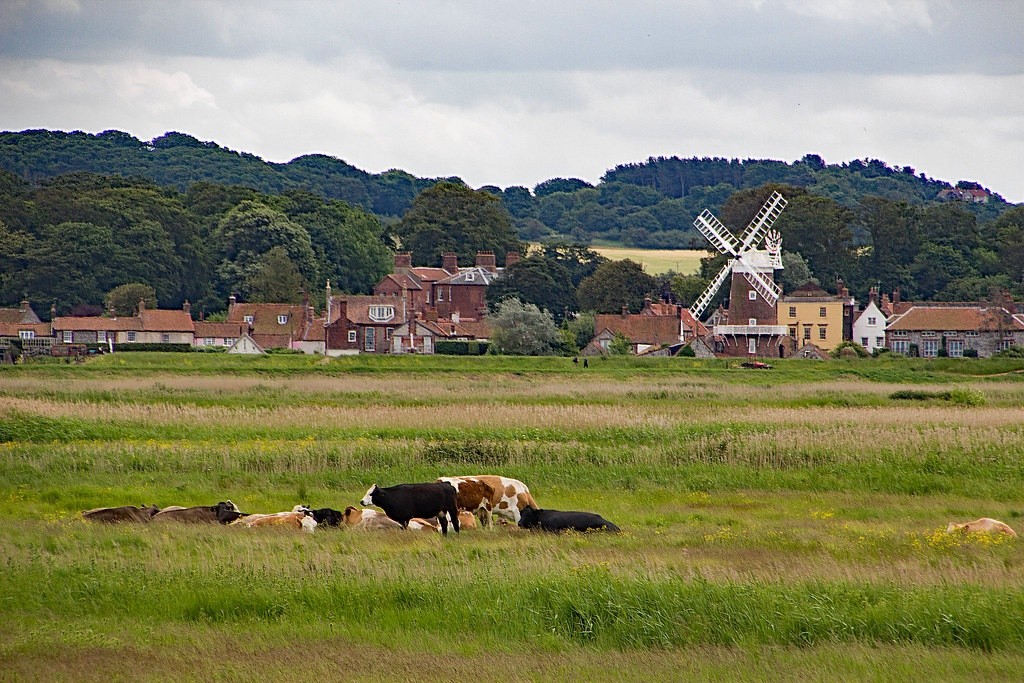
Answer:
[779,344,786,359]
[583,359,588,369]
[573,357,578,366]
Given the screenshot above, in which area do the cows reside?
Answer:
[516,506,621,534]
[80,474,539,530]
[946,517,1019,540]
